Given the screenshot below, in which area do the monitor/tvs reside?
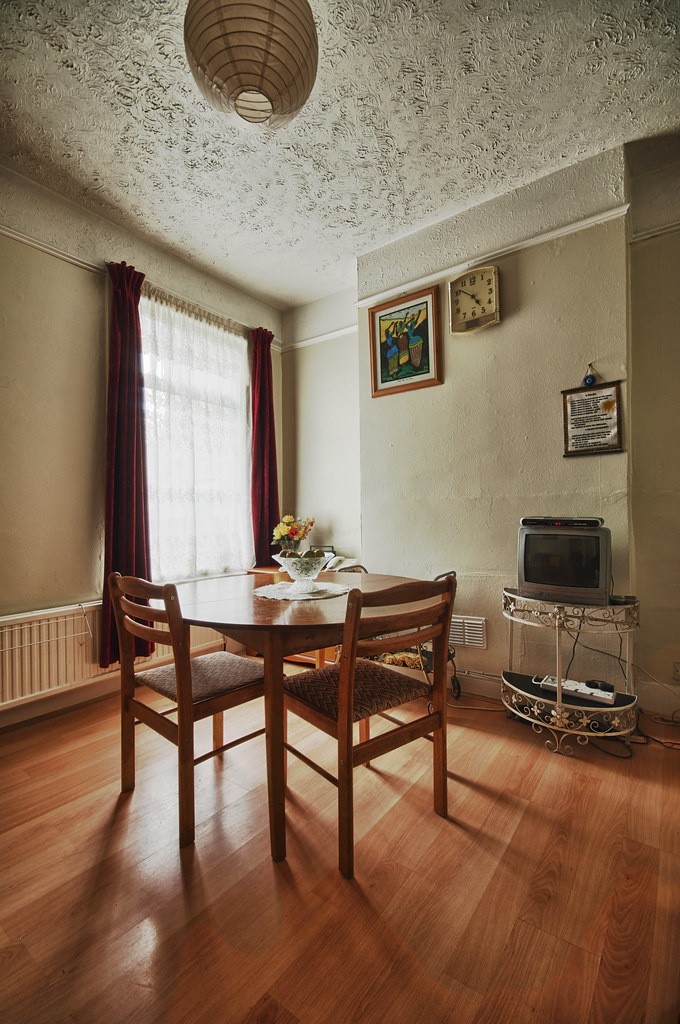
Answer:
[517,526,611,606]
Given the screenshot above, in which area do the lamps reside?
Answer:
[184,1,318,133]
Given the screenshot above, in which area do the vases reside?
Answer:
[280,541,300,552]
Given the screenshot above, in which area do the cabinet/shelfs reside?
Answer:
[501,587,639,757]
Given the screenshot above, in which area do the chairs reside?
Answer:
[108,572,289,847]
[284,576,456,878]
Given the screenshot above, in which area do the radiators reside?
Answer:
[0,588,224,707]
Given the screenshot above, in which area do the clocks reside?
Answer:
[449,265,500,336]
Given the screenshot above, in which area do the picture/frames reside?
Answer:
[368,285,443,398]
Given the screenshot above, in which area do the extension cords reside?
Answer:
[540,675,616,705]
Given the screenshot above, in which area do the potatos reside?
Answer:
[279,548,325,558]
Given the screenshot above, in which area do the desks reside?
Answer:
[149,572,445,862]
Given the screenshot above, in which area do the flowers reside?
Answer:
[271,515,315,545]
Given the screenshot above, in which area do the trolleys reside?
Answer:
[336,564,462,714]
